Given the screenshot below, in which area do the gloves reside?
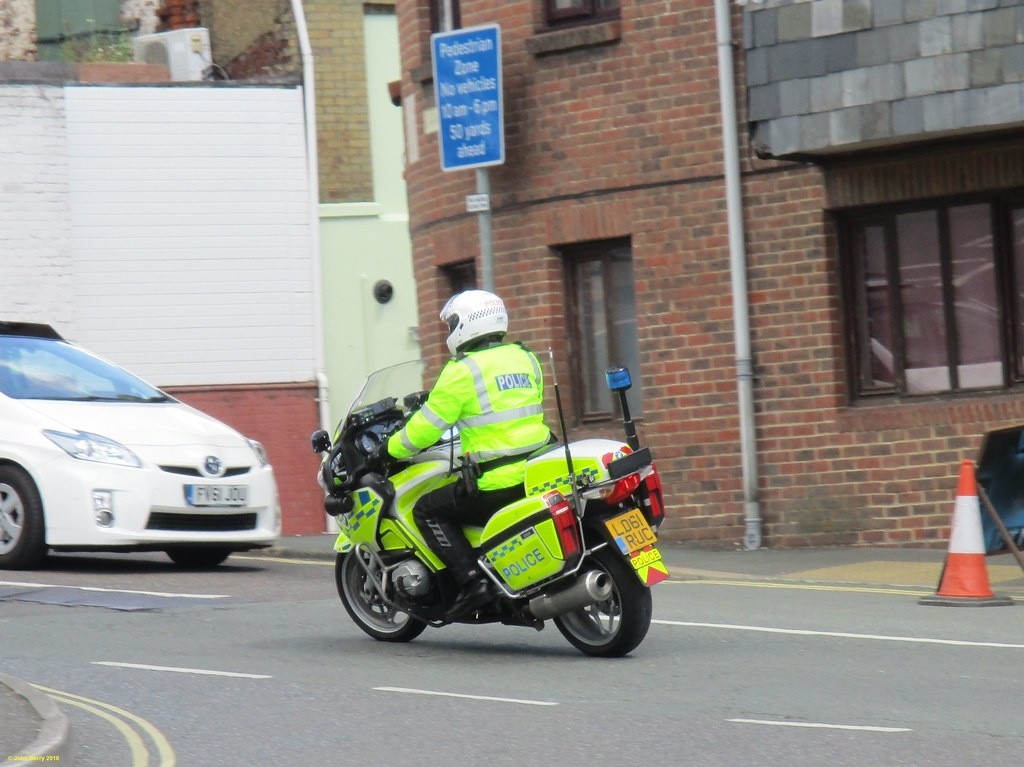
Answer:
[362,436,393,468]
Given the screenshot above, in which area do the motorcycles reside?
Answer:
[312,353,670,655]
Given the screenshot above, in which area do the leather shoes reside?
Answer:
[444,576,494,619]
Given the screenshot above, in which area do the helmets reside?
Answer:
[440,289,508,358]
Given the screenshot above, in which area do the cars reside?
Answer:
[2,336,280,564]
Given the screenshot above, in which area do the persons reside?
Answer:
[375,289,557,611]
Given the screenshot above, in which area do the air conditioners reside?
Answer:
[132,26,216,83]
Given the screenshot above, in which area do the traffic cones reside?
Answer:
[920,456,1015,606]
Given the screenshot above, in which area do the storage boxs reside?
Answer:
[481,491,585,592]
